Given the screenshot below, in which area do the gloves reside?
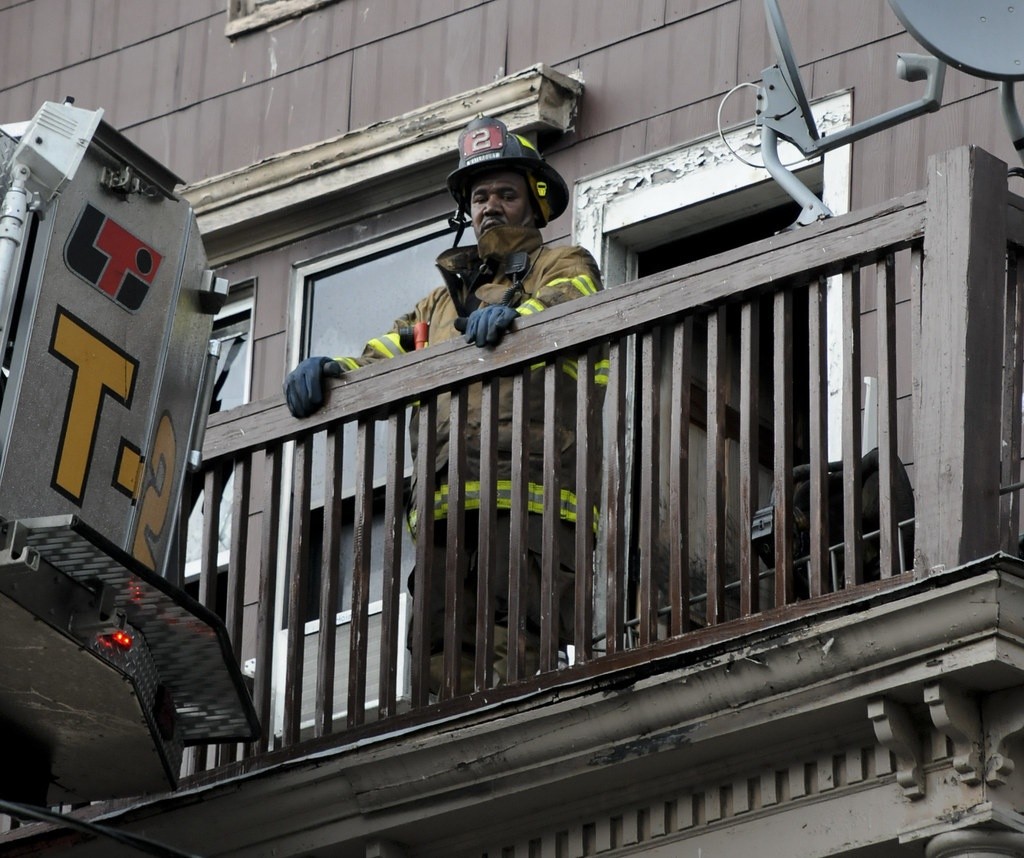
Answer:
[454,305,521,348]
[282,356,341,419]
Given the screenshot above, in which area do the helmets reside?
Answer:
[446,113,569,222]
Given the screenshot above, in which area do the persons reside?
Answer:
[283,112,610,704]
